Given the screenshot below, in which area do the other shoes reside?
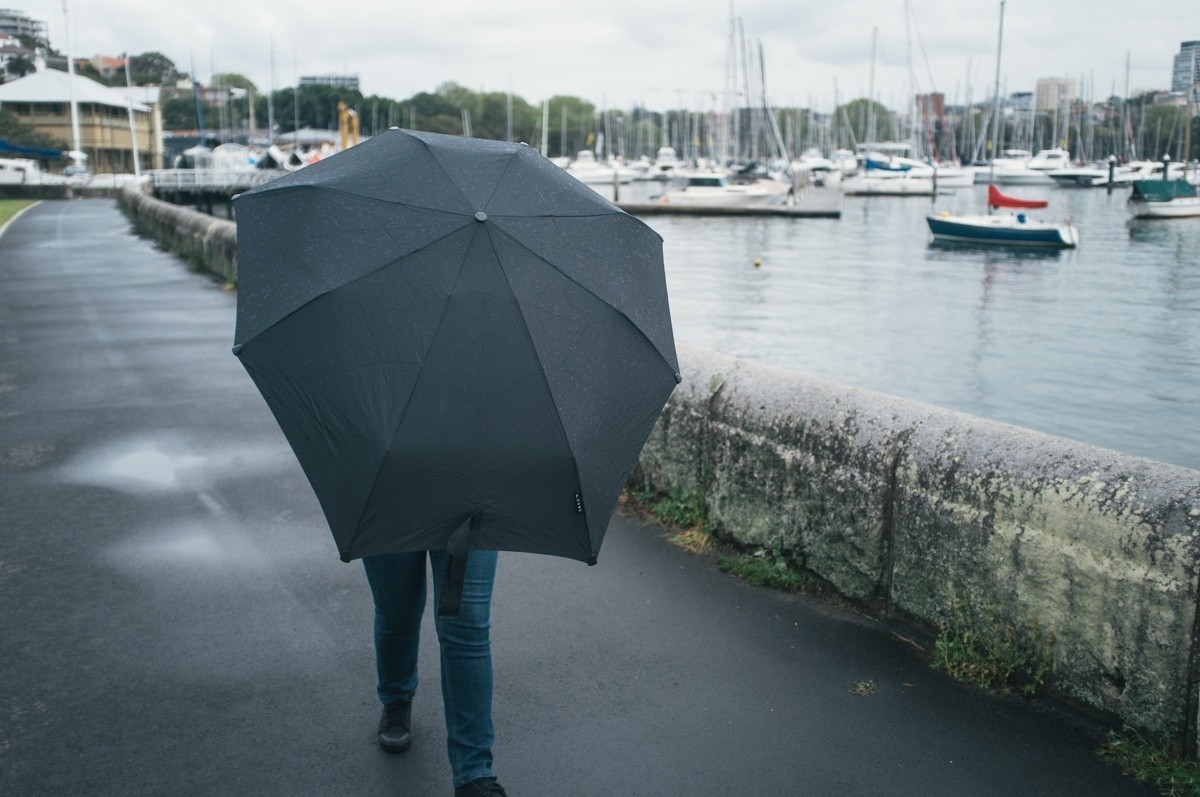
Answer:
[377,702,412,751]
[454,776,506,797]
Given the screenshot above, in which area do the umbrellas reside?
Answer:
[234,126,682,568]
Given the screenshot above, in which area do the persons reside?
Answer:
[359,551,505,797]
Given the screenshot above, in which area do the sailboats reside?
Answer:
[924,0,1080,247]
[175,0,1200,217]
